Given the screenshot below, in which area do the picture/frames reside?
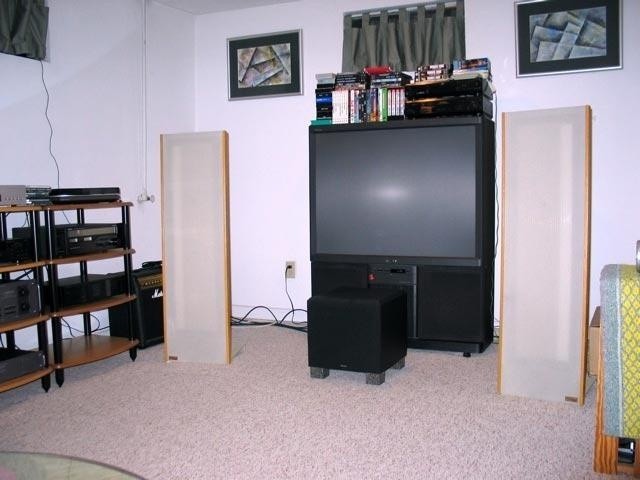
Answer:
[226,29,303,101]
[514,0,622,77]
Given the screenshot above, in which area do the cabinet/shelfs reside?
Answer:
[309,261,493,356]
[593,261,640,476]
[0,202,140,392]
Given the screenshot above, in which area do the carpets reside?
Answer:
[0,450,143,479]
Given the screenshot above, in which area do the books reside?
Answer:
[311,58,495,127]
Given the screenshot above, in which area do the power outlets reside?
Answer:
[285,261,295,279]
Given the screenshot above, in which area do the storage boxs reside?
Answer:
[586,306,601,378]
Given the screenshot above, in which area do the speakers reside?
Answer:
[108,268,163,348]
[311,263,368,295]
[418,268,491,341]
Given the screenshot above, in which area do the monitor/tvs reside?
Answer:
[309,116,494,265]
